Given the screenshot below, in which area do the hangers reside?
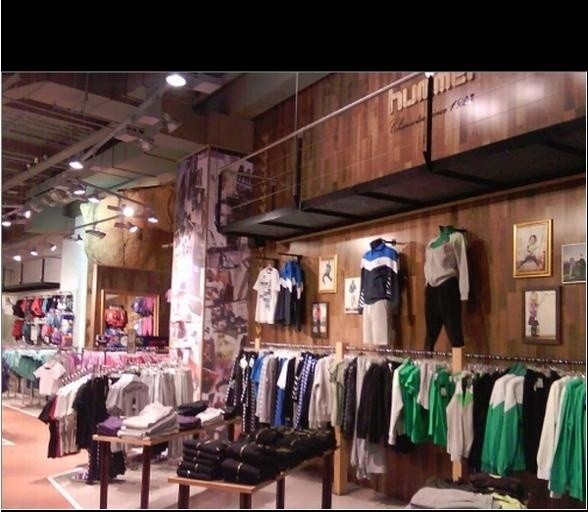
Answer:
[42,346,189,388]
[262,249,300,271]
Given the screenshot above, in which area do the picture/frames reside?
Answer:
[520,283,564,346]
[559,241,586,285]
[344,276,363,315]
[317,252,339,294]
[311,301,330,339]
[512,215,554,281]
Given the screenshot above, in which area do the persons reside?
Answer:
[314,303,320,335]
[348,280,357,308]
[357,239,399,347]
[514,235,542,271]
[527,293,540,339]
[422,224,469,350]
[321,259,332,285]
[568,254,585,280]
[205,195,253,303]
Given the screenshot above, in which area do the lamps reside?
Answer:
[10,210,160,261]
[1,182,76,230]
[62,173,157,216]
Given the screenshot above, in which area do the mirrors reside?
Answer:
[99,288,161,340]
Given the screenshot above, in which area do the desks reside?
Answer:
[91,416,242,508]
[167,450,335,509]
